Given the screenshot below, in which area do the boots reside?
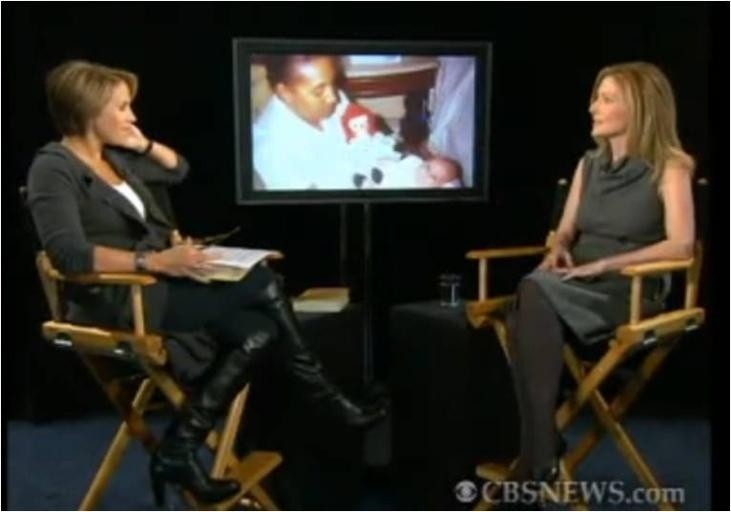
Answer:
[263,288,385,425]
[151,349,251,511]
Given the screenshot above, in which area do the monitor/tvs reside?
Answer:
[229,36,495,206]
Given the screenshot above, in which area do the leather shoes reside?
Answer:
[489,442,566,511]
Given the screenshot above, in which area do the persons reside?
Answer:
[27,60,390,510]
[484,62,702,511]
[383,153,462,191]
[338,103,397,186]
[252,54,408,193]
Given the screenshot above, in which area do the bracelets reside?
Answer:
[140,139,154,157]
[134,246,153,273]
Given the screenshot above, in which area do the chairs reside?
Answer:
[16,184,285,510]
[464,176,708,509]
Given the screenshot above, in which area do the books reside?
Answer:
[294,287,350,314]
[191,243,285,285]
